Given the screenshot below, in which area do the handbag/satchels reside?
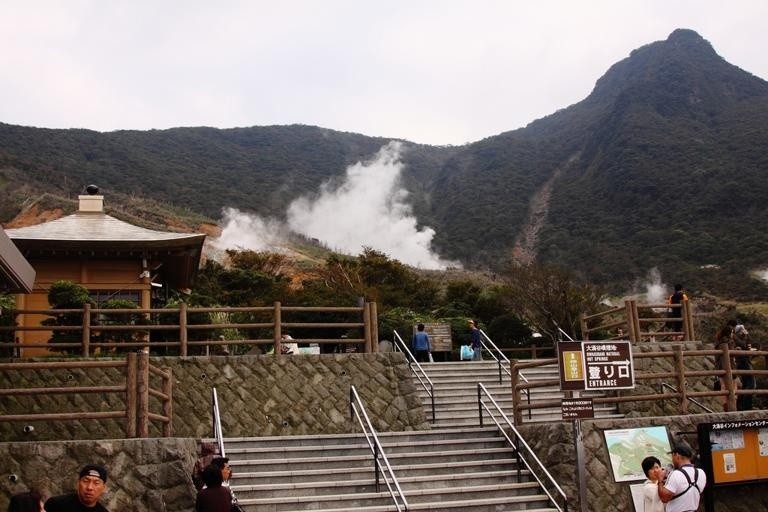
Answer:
[713,375,722,390]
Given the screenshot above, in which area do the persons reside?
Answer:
[196,465,232,512]
[201,457,238,505]
[281,328,301,357]
[712,317,759,413]
[7,489,47,511]
[465,318,485,360]
[668,283,690,339]
[657,444,711,512]
[411,322,433,362]
[42,465,110,512]
[639,456,666,511]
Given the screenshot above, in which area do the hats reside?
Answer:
[78,463,107,484]
[666,446,694,459]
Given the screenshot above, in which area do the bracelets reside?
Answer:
[657,480,664,484]
